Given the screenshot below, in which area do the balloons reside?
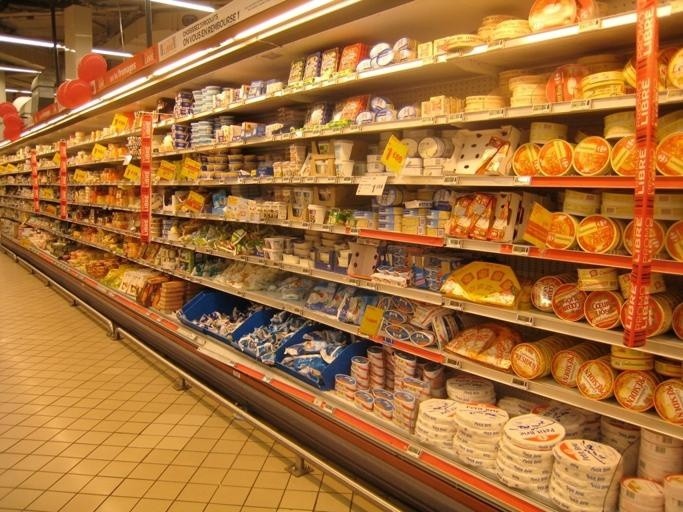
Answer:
[77,52,107,83]
[3,126,21,140]
[56,80,69,105]
[4,113,23,129]
[0,102,19,118]
[64,80,92,108]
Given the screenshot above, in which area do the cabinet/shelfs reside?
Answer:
[0,0,683,512]
